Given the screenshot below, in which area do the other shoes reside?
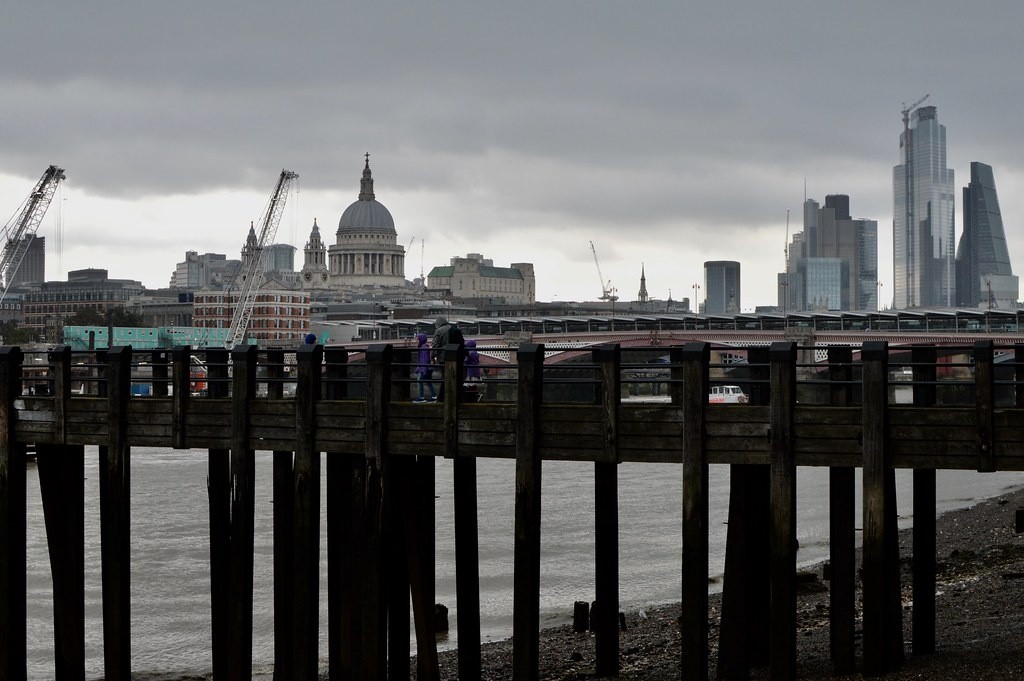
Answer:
[476,394,482,402]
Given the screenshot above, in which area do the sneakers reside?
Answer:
[426,397,438,402]
[412,398,426,403]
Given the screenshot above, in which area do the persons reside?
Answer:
[306,334,316,343]
[431,317,468,401]
[413,333,437,402]
[464,340,483,402]
